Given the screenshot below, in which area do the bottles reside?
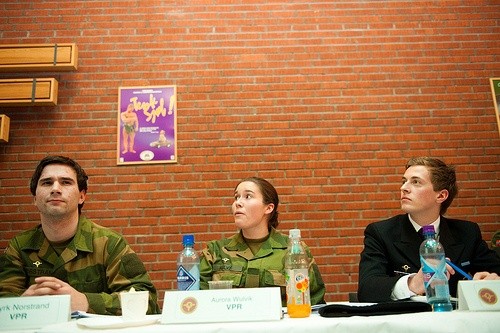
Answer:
[419,225,453,313]
[283,229,311,318]
[176,234,200,291]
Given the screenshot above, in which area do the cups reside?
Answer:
[119,291,149,320]
[209,281,232,289]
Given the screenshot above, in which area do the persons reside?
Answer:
[356,155,500,303]
[0,154,160,316]
[198,177,326,307]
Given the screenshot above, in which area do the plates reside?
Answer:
[76,314,158,329]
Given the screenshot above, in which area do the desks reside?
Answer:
[0,301,500,333]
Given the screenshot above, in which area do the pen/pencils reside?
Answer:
[444,259,473,280]
